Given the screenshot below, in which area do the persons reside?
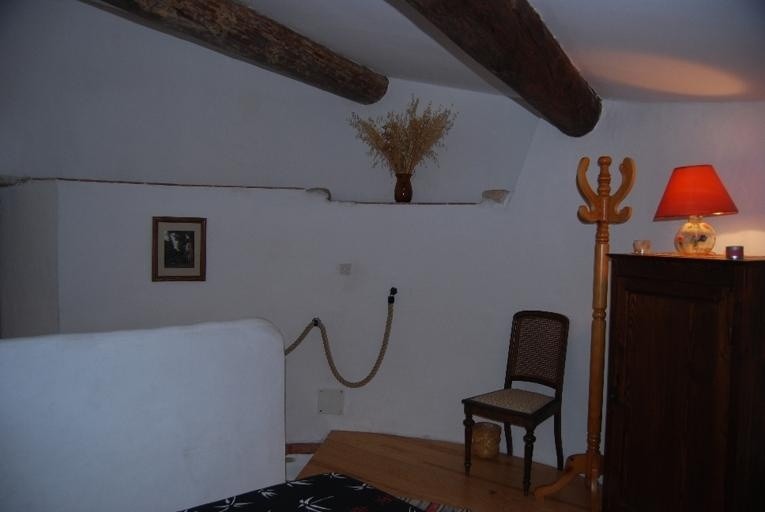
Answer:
[165,232,194,268]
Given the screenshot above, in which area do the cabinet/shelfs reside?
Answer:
[602,252,765,511]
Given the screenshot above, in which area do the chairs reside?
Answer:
[461,310,571,496]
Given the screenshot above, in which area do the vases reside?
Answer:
[393,173,413,203]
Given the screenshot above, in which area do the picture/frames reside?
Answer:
[150,213,208,282]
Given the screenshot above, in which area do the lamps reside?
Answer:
[652,163,738,258]
